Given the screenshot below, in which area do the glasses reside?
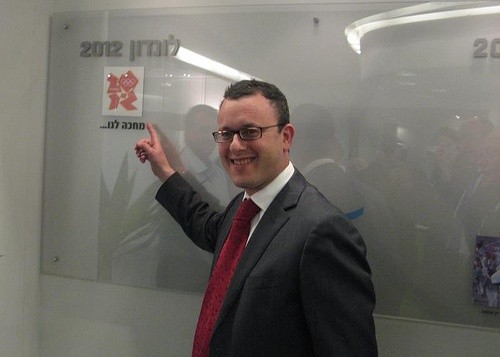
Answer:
[212,125,278,143]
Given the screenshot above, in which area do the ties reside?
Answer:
[191,198,261,357]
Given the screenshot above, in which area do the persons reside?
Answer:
[135,80,379,357]
[171,103,386,315]
